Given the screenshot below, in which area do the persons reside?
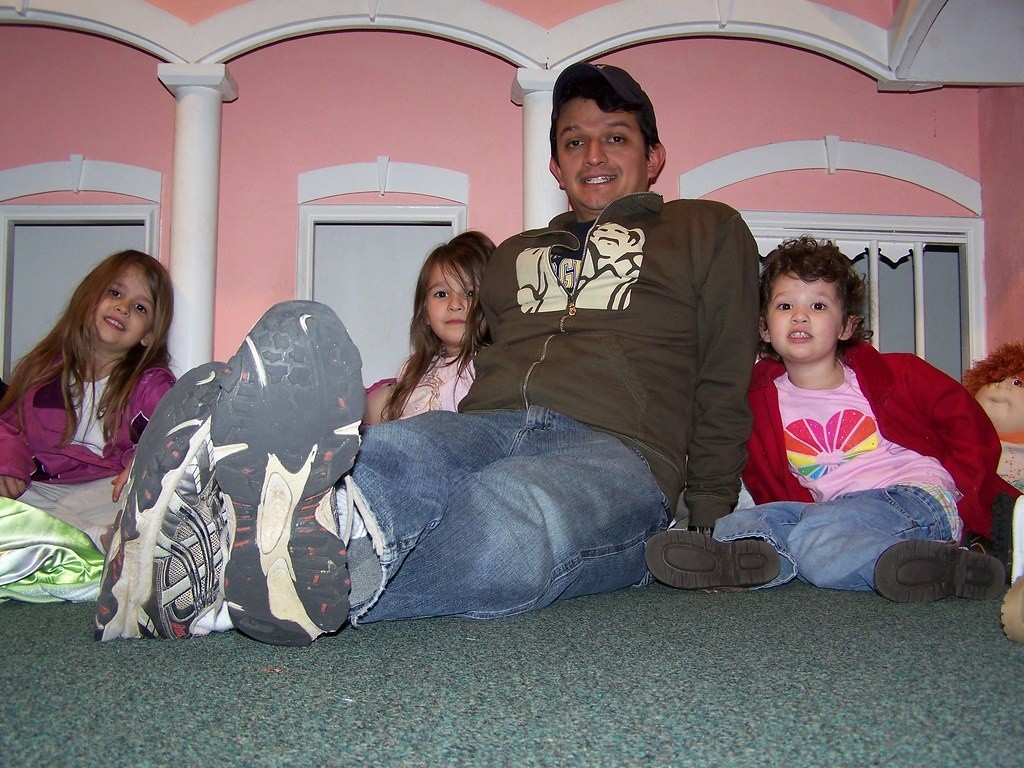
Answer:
[91,63,762,619]
[646,234,1024,643]
[362,230,498,429]
[0,249,177,538]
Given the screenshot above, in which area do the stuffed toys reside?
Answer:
[962,341,1024,493]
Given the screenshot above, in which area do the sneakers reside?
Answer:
[94,361,236,642]
[211,300,365,648]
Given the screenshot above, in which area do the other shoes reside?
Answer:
[874,540,1007,603]
[645,530,781,589]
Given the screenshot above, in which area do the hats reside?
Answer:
[550,62,656,123]
